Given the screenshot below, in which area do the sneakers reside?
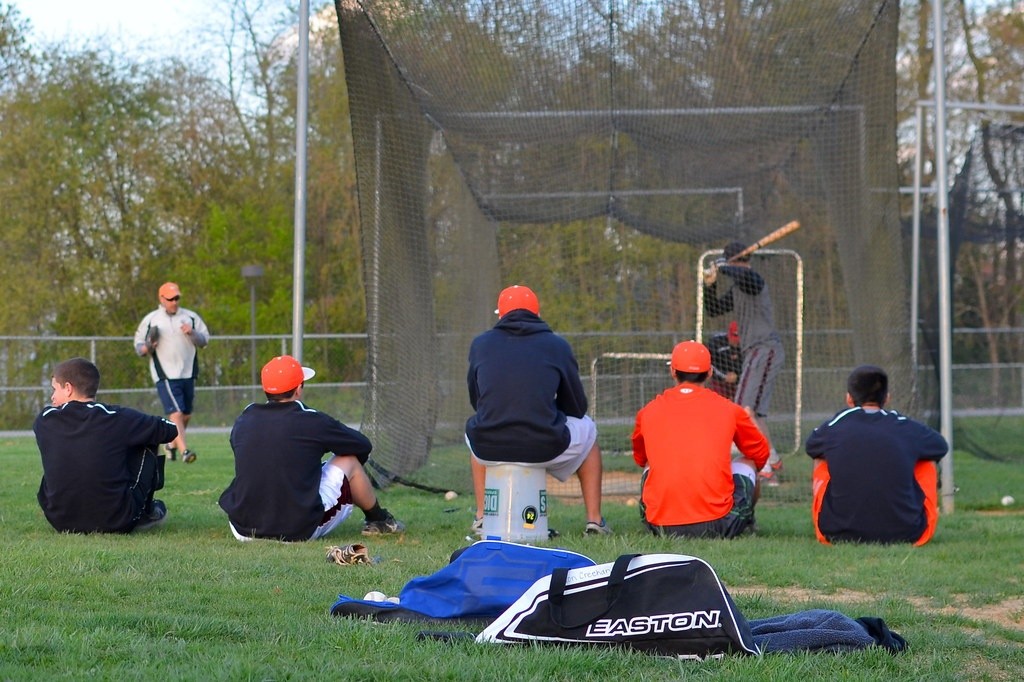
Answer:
[129,500,168,534]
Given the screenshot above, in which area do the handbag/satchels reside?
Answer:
[473,553,758,662]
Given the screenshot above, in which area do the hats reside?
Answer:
[494,285,539,319]
[667,339,711,373]
[261,355,315,395]
[159,281,181,300]
[727,322,740,343]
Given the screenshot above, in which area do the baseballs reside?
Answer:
[363,591,389,602]
[445,491,458,500]
[1001,495,1015,505]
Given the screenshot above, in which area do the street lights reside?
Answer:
[242,265,263,401]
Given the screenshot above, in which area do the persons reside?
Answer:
[702,242,784,486]
[705,322,744,455]
[217,355,405,543]
[805,365,948,547]
[135,282,210,462]
[33,356,178,534]
[632,341,770,540]
[464,286,612,542]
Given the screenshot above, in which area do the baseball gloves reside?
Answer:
[325,544,372,565]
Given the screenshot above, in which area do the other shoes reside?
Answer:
[586,518,612,535]
[470,516,482,531]
[163,443,177,462]
[182,449,196,464]
[362,509,406,537]
[757,457,784,487]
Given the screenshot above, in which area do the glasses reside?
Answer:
[167,295,180,301]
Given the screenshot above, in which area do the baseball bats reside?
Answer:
[703,220,801,278]
